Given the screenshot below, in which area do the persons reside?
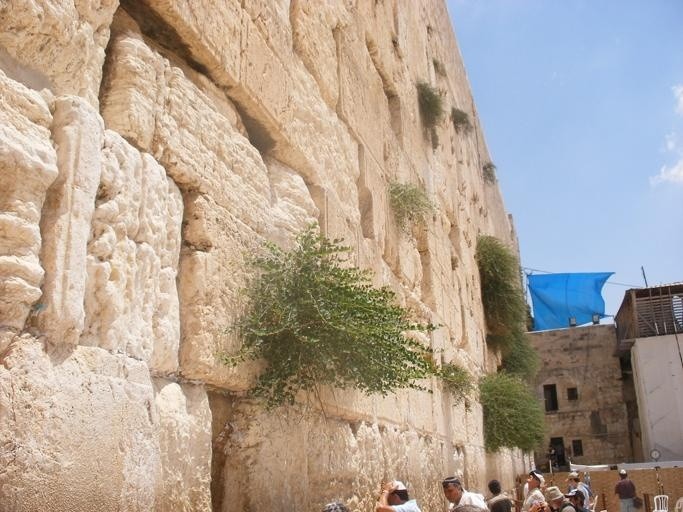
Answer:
[613,469,636,512]
[371,470,597,512]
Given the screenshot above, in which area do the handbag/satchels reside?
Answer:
[561,503,591,512]
[633,498,642,507]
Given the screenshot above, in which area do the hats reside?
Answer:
[545,486,563,501]
[395,481,406,491]
[564,489,584,499]
[531,470,545,488]
[565,473,580,482]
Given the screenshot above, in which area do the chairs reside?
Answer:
[652,494,682,511]
